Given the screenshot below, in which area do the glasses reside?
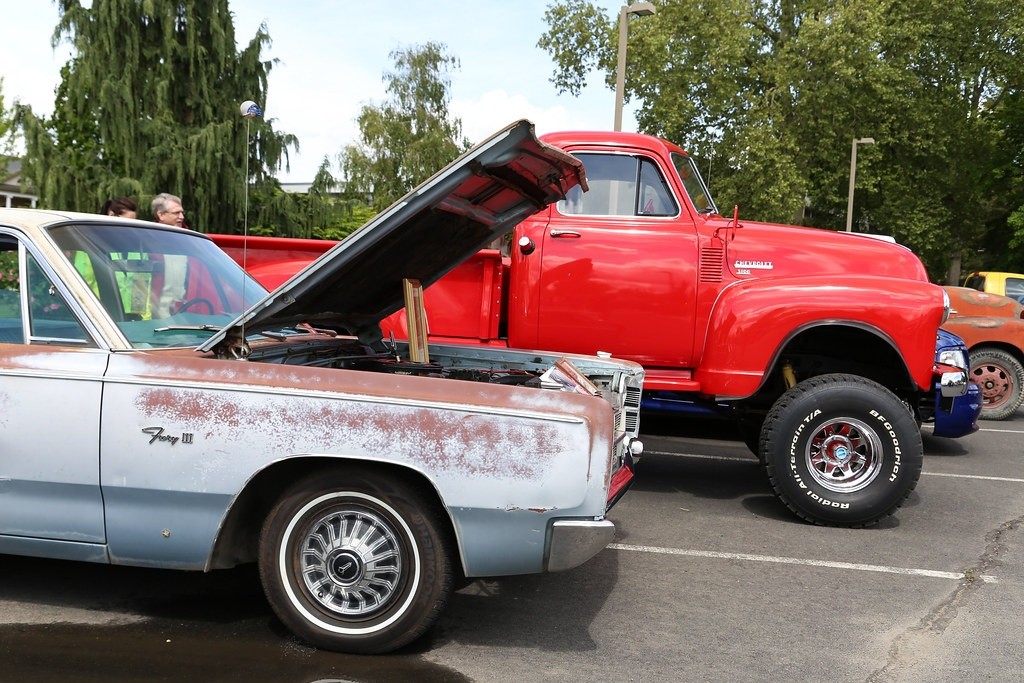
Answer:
[165,210,185,215]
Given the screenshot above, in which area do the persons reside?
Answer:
[74,197,151,321]
[149,193,188,320]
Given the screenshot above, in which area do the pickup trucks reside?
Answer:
[187,131,949,528]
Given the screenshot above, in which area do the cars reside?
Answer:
[0,115,644,654]
[634,327,982,437]
[963,272,1024,305]
[941,284,1024,420]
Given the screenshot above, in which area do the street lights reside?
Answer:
[846,137,875,232]
[610,2,655,213]
